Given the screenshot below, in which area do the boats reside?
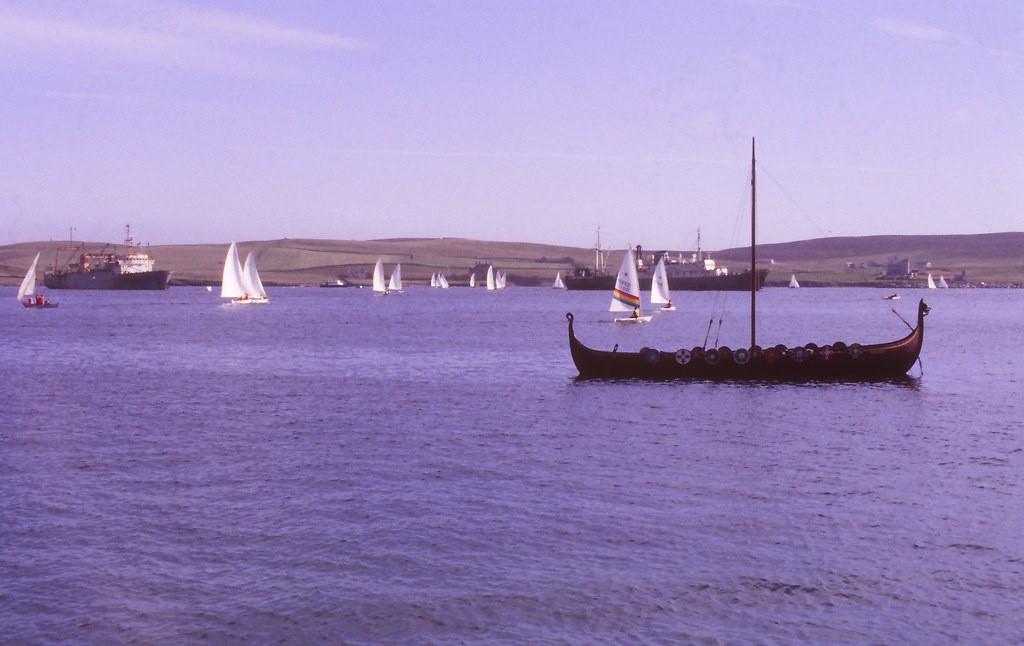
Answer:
[884,296,900,300]
[565,227,772,292]
[566,136,931,380]
[320,280,346,288]
[44,224,174,290]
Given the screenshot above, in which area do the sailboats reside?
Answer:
[496,271,506,288]
[789,275,800,288]
[220,241,268,305]
[18,252,59,308]
[469,273,475,287]
[608,246,653,323]
[937,275,948,288]
[431,272,449,289]
[552,272,564,288]
[928,274,936,289]
[372,258,389,295]
[487,265,494,290]
[388,264,404,293]
[650,256,676,311]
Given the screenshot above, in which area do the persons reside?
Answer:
[667,300,672,308]
[633,307,639,317]
[29,294,44,305]
